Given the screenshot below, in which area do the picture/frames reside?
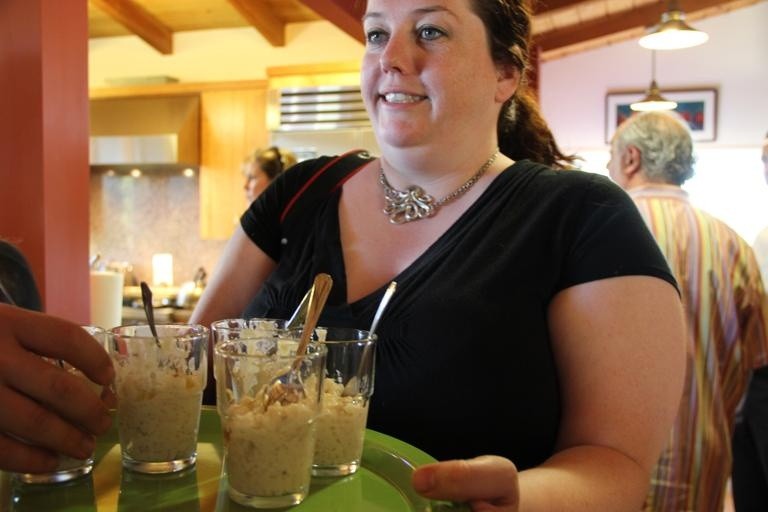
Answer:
[603,86,719,144]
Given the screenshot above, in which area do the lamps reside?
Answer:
[638,0,709,52]
[629,49,678,113]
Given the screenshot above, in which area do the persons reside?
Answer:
[0,299,115,476]
[174,1,685,511]
[606,112,766,512]
[240,146,298,206]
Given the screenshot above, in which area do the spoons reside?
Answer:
[263,273,398,414]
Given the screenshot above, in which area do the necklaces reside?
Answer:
[368,141,503,226]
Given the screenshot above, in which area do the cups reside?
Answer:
[25,326,102,481]
[108,324,209,477]
[208,318,303,440]
[309,331,374,478]
[216,338,328,506]
